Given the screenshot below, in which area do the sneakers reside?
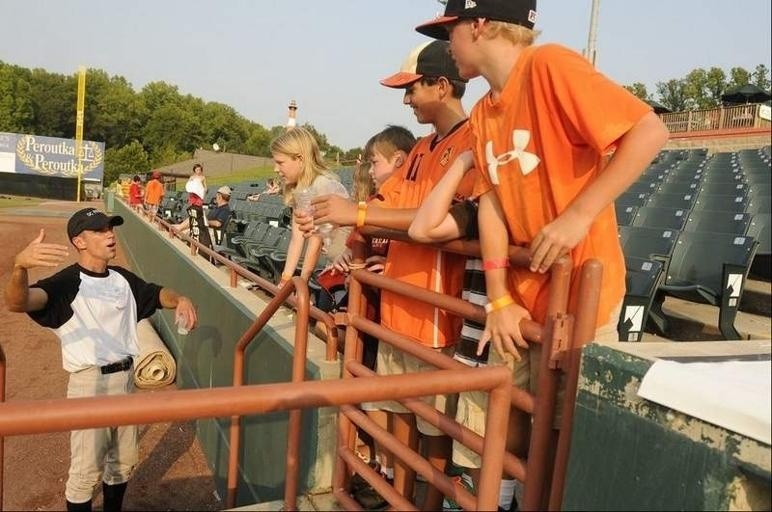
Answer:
[349,460,382,495]
[354,474,394,510]
[441,476,476,512]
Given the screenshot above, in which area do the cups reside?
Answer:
[291,190,339,234]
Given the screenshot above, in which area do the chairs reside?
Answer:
[155,167,356,286]
[614,145,772,342]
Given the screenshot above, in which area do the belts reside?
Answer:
[101,356,135,376]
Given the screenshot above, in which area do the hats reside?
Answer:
[414,0,537,42]
[378,38,469,89]
[151,171,161,179]
[216,185,233,195]
[66,207,125,240]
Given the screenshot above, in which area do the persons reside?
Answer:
[117,172,164,216]
[185,164,207,208]
[5,208,199,512]
[172,186,232,246]
